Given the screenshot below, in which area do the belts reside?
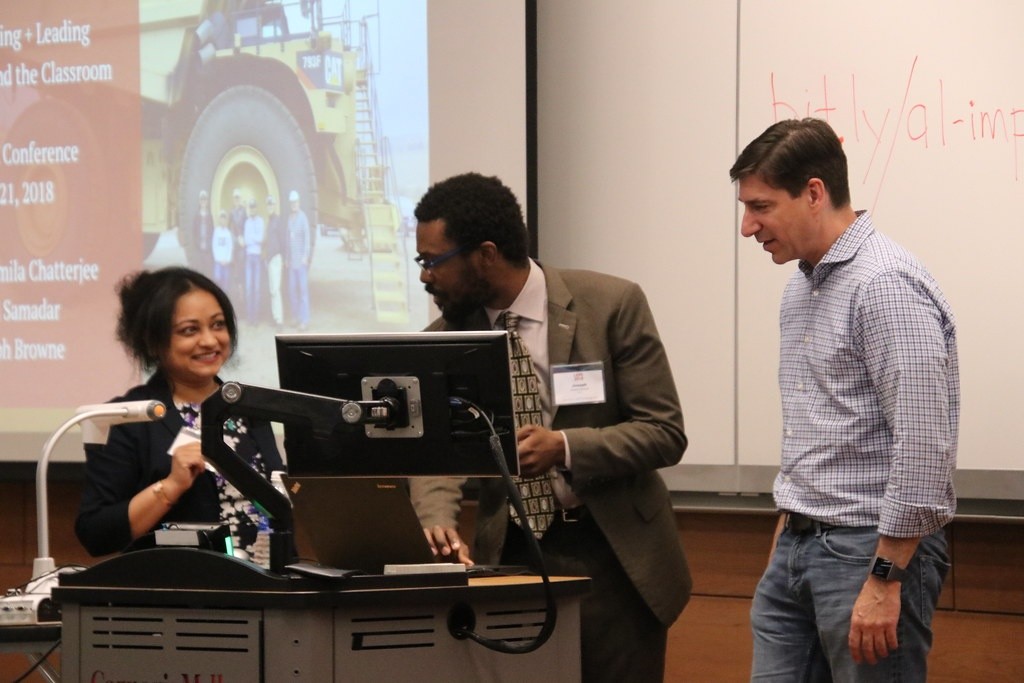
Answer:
[785,509,834,534]
[555,506,587,524]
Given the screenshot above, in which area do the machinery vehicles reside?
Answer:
[138,0,427,332]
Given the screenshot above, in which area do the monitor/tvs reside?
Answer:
[274,330,521,478]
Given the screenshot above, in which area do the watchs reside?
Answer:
[870,556,906,583]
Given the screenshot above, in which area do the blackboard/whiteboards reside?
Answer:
[537,1,1024,519]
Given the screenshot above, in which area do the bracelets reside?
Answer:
[151,481,173,508]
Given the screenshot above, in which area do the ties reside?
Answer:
[500,311,554,541]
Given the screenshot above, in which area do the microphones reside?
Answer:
[24,398,168,595]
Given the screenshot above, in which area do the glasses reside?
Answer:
[415,242,472,275]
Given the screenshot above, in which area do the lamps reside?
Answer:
[0,398,167,626]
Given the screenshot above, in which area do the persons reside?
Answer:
[194,190,312,332]
[73,267,283,551]
[732,119,961,683]
[405,174,688,683]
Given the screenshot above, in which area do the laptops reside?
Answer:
[280,471,536,579]
